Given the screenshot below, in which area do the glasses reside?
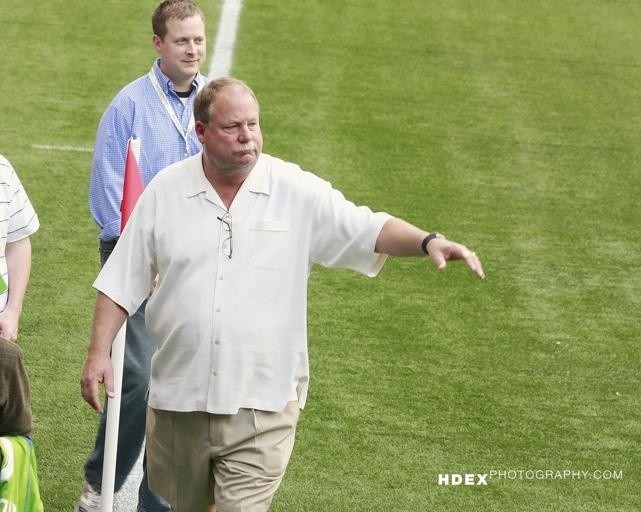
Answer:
[216,210,234,262]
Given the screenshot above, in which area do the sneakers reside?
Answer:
[78,479,101,512]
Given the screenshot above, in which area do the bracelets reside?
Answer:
[420,232,443,256]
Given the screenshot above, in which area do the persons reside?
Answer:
[0,337,45,512]
[80,75,487,512]
[0,151,41,345]
[75,0,208,512]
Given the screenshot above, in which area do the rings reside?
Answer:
[465,251,475,260]
